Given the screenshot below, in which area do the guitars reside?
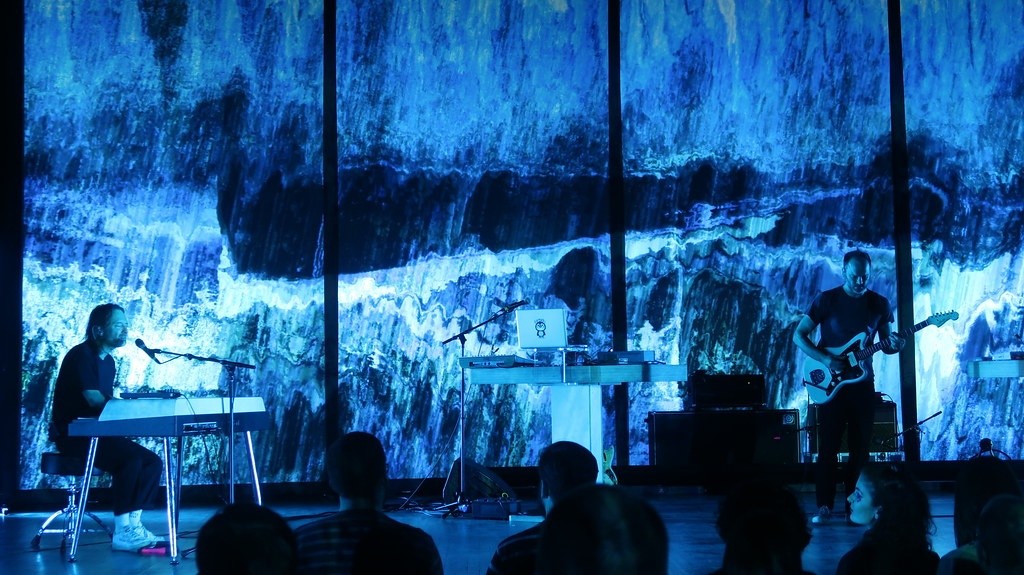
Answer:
[803,308,960,405]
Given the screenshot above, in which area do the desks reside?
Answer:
[468,365,687,484]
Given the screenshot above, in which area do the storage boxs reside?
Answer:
[810,401,898,452]
[649,409,800,467]
[470,497,522,519]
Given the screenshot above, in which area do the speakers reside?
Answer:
[807,402,898,453]
[686,372,769,410]
[646,408,802,466]
[443,456,517,517]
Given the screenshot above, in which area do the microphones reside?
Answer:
[135,339,161,365]
[501,297,531,311]
[873,437,895,449]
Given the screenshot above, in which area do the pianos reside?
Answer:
[64,395,274,568]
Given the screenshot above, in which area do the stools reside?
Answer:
[31,453,114,549]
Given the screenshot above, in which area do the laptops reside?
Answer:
[515,309,588,349]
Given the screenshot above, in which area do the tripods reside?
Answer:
[434,312,512,519]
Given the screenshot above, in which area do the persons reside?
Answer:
[52,303,163,553]
[484,442,603,575]
[793,251,907,525]
[294,428,445,575]
[704,451,815,575]
[834,461,1024,575]
[541,484,668,575]
[193,504,301,575]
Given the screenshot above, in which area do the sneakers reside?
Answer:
[112,527,157,551]
[812,504,831,523]
[131,524,165,543]
